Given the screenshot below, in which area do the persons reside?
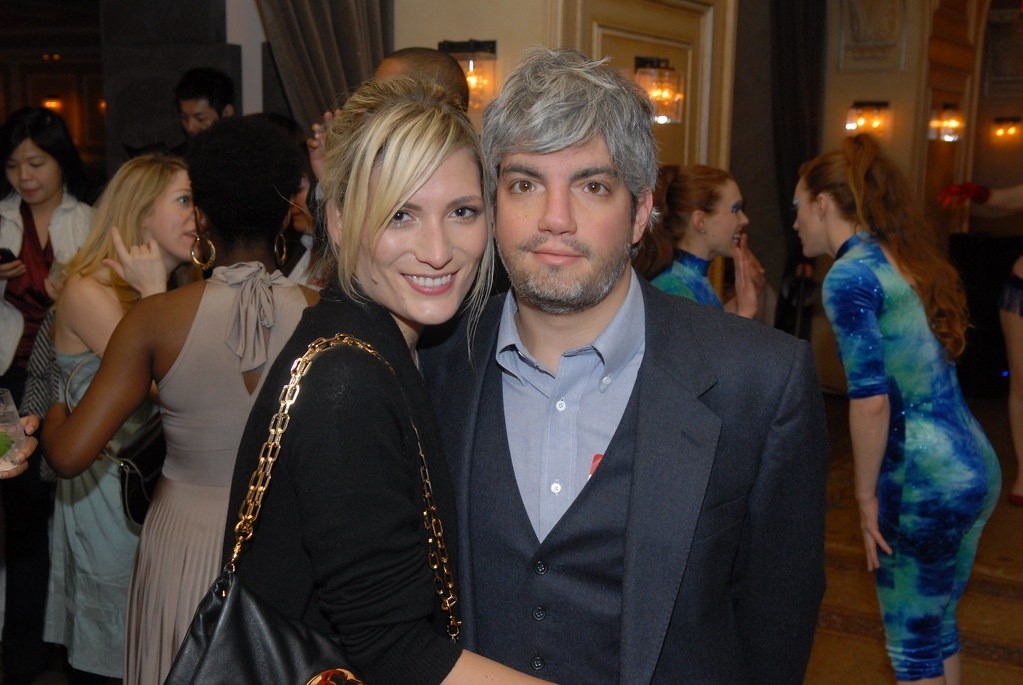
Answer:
[793,134,1004,685]
[0,46,469,685]
[418,46,824,685]
[646,165,776,324]
[222,75,560,685]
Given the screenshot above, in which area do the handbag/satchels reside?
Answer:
[64,351,166,529]
[163,333,459,685]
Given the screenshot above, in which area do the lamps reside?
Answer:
[632,53,684,125]
[437,38,497,139]
[844,98,1023,143]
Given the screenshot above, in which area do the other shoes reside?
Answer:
[1008,490,1023,506]
